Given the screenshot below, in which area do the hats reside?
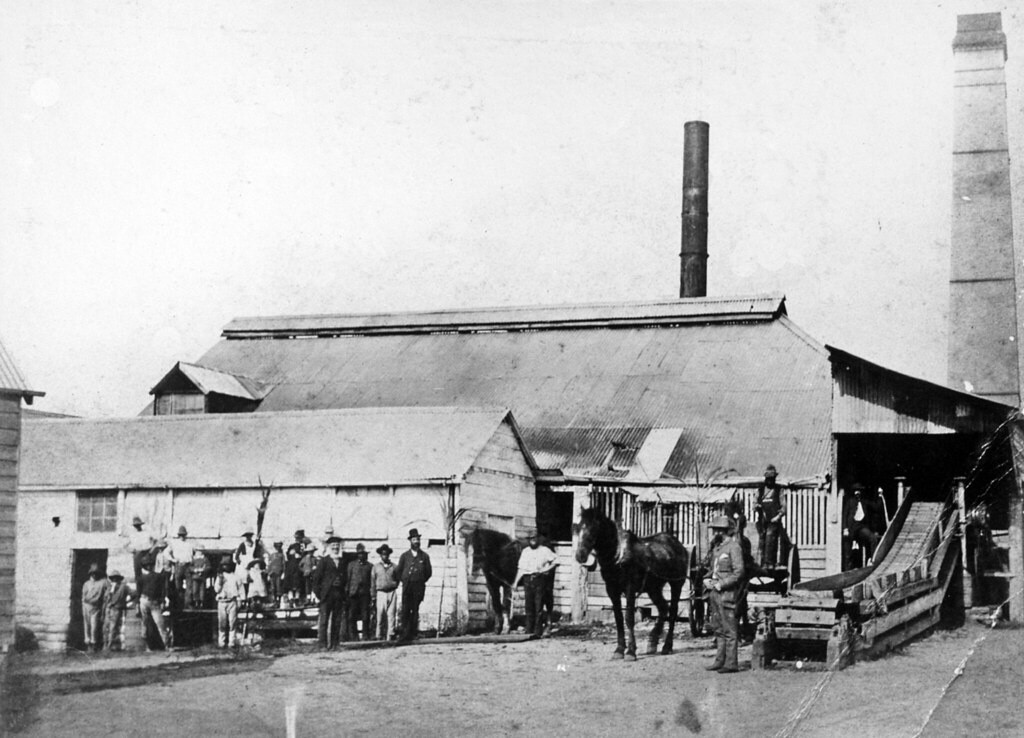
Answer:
[525,527,538,537]
[140,554,153,567]
[325,536,344,544]
[247,557,265,570]
[707,516,736,528]
[87,562,103,575]
[376,544,393,554]
[241,531,255,537]
[407,529,421,539]
[196,544,204,551]
[355,544,370,556]
[156,540,170,548]
[220,556,234,566]
[109,570,123,582]
[132,516,144,526]
[177,525,187,536]
[764,465,779,476]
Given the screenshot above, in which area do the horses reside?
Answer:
[462,524,557,637]
[573,504,689,662]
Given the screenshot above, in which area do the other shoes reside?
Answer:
[761,563,774,570]
[705,665,740,673]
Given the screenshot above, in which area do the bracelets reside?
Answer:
[779,513,782,517]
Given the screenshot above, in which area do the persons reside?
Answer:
[82,517,433,655]
[513,531,560,640]
[705,517,746,672]
[752,465,787,570]
[842,483,878,571]
